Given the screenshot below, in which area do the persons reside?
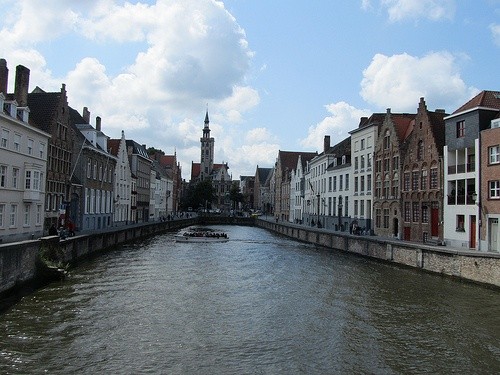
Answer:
[184,232,227,237]
[349,222,364,235]
[160,212,193,222]
[294,218,322,228]
[49,220,77,239]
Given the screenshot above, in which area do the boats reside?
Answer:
[173,230,228,242]
